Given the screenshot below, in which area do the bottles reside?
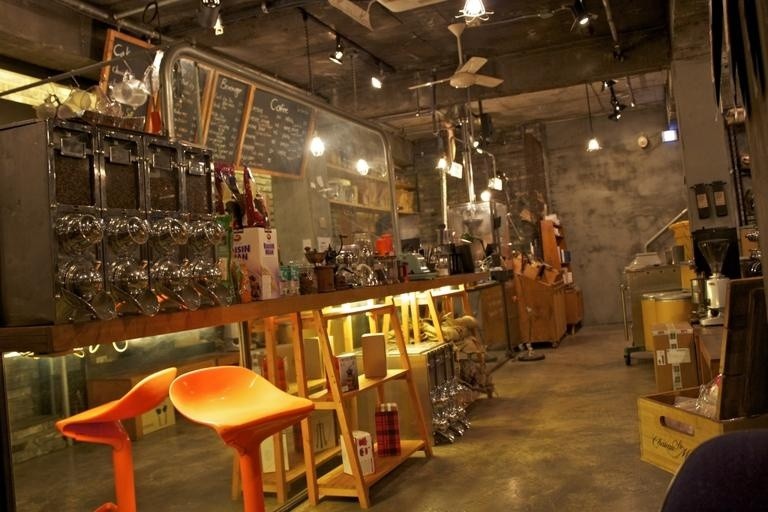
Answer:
[374,402,401,457]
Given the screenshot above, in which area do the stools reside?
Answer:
[55,367,177,512]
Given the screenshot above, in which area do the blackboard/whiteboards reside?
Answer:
[98,28,317,180]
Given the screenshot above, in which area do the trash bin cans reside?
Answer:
[641,289,692,353]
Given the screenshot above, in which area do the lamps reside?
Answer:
[585,82,604,153]
[602,79,628,122]
[296,6,395,89]
[570,0,598,30]
[455,0,495,25]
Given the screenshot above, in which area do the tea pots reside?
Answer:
[113,71,148,110]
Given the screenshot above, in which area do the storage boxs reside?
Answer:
[637,276,768,475]
[85,352,239,438]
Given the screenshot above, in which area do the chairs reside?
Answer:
[169,366,315,512]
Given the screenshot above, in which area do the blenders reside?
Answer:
[698,238,730,326]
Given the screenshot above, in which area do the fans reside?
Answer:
[408,23,504,89]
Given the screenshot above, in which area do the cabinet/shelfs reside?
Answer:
[236,316,359,505]
[382,296,446,350]
[410,284,493,400]
[540,220,584,336]
[291,297,434,509]
